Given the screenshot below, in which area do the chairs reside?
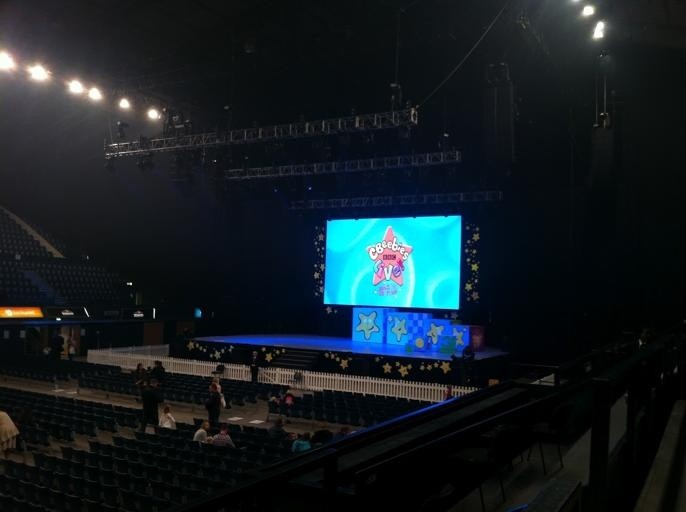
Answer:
[0,197,143,358]
[2,358,447,511]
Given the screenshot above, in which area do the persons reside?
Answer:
[136,359,351,455]
[444,386,455,400]
